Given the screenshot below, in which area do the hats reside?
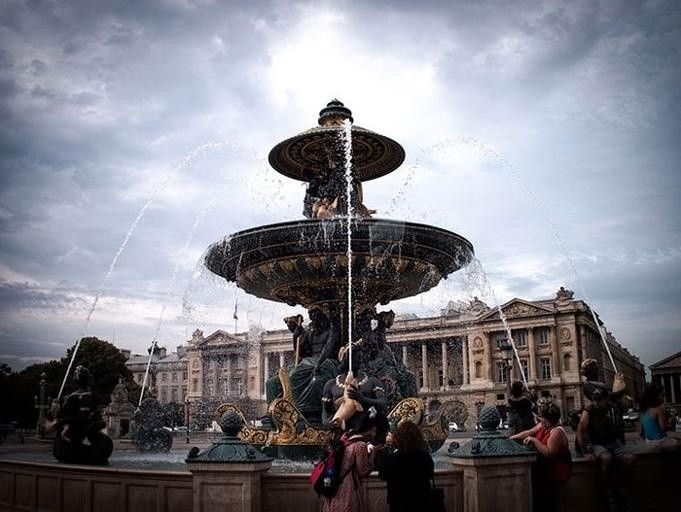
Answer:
[591,386,609,401]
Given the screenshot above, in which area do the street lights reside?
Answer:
[148,340,166,400]
[500,334,512,429]
[34,372,52,435]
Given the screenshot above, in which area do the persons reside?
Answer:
[287,305,342,420]
[375,419,436,511]
[508,379,538,434]
[378,310,421,399]
[266,314,311,406]
[316,410,382,511]
[580,355,626,402]
[509,403,574,512]
[640,387,669,439]
[573,386,636,511]
[321,338,391,446]
[49,361,117,466]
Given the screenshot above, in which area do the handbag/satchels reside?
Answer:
[429,476,447,512]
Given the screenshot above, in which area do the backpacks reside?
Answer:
[309,428,367,499]
